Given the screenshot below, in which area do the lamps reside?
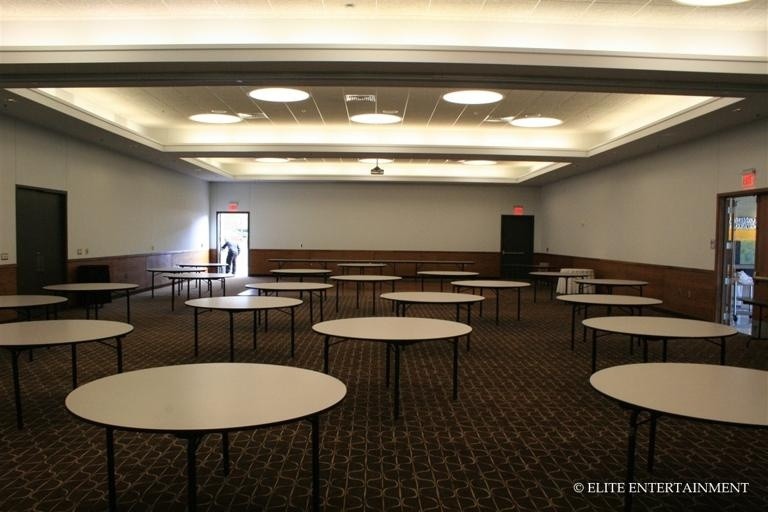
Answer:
[370,156,384,175]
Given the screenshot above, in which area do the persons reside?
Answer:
[221,236,240,274]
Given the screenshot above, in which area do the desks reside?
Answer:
[44,281,139,327]
[574,278,645,315]
[0,320,135,432]
[269,267,332,301]
[146,266,208,300]
[185,296,304,364]
[449,280,530,326]
[64,363,347,512]
[163,273,235,312]
[582,315,739,373]
[416,270,480,300]
[336,263,388,292]
[556,293,662,351]
[311,317,474,418]
[328,274,403,315]
[0,293,66,359]
[379,292,485,352]
[179,263,230,290]
[528,272,589,303]
[245,282,334,334]
[590,362,768,506]
